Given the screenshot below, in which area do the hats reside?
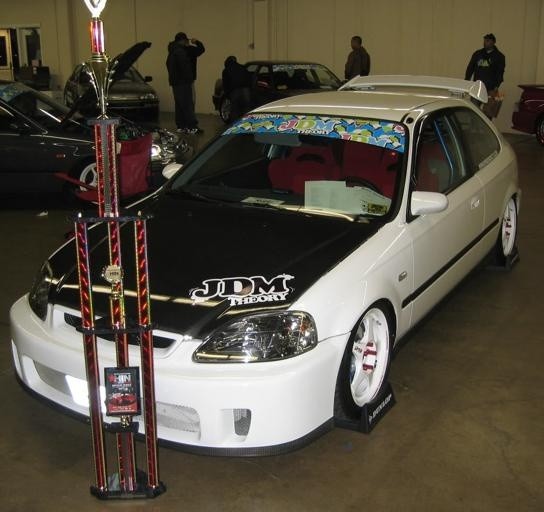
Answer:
[175,32,192,40]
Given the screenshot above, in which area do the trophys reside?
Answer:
[66,0,167,502]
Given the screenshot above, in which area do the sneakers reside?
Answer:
[175,127,201,136]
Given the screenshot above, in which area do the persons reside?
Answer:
[463,31,507,123]
[183,38,199,124]
[343,35,371,85]
[165,30,206,136]
[220,55,252,124]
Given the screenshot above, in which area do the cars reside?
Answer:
[9,74,523,459]
[62,61,160,123]
[211,59,349,125]
[0,78,190,211]
[510,83,544,146]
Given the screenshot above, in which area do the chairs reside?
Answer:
[268,136,437,210]
[260,69,307,87]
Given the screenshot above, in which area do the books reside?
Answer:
[352,185,393,217]
[304,180,349,214]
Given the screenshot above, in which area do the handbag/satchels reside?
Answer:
[480,87,505,119]
